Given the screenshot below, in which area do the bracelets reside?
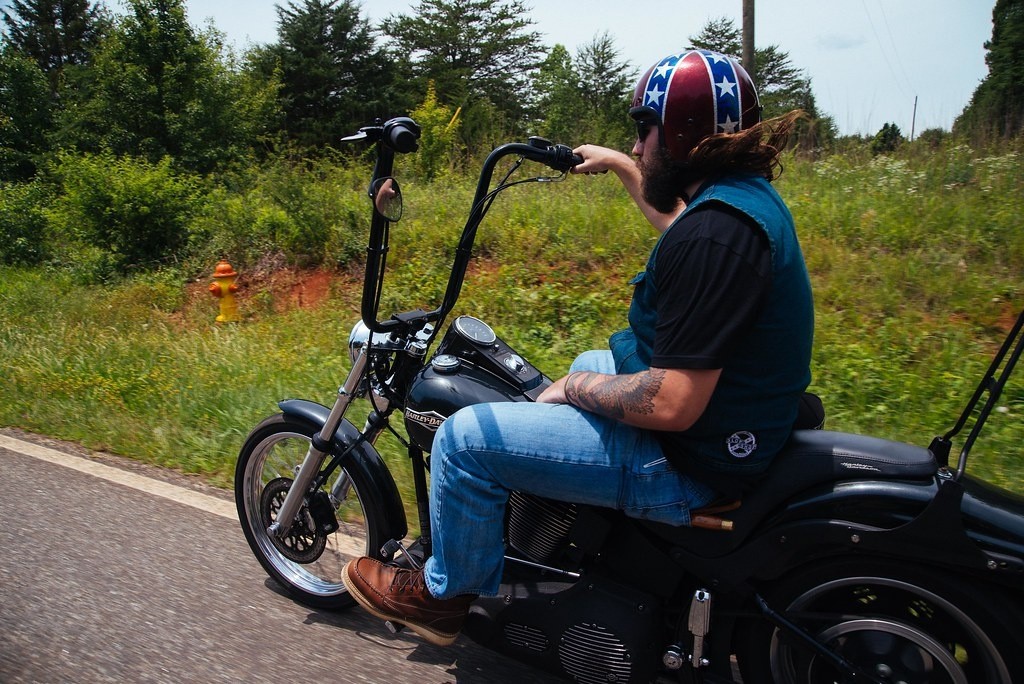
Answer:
[564,371,583,407]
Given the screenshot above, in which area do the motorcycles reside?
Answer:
[233,118,1024,684]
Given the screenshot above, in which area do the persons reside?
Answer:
[342,49,814,650]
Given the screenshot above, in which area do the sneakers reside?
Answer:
[340,557,475,648]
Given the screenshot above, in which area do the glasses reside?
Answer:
[635,119,658,142]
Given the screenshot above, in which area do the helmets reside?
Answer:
[630,48,763,168]
